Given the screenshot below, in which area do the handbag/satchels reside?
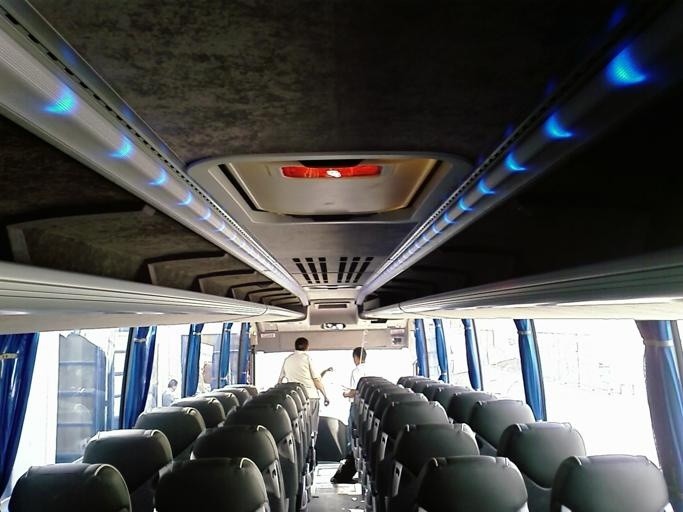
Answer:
[331,457,357,484]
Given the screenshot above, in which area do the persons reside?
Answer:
[342,345,372,457]
[277,336,329,441]
[161,378,178,408]
[191,367,207,397]
